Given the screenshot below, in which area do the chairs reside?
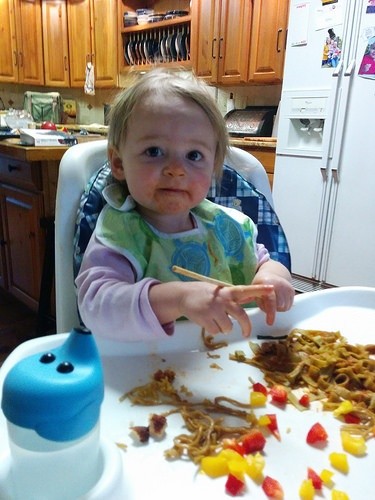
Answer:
[53,141,291,336]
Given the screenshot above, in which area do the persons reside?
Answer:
[321,38,338,68]
[75,67,295,337]
[359,42,375,74]
[366,0,375,14]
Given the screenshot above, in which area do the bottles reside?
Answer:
[2,327,104,500]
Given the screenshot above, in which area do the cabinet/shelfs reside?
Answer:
[1,155,64,327]
[244,148,275,190]
[0,1,289,87]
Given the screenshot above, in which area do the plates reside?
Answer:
[124,24,190,61]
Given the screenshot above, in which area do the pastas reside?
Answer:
[119,328,375,464]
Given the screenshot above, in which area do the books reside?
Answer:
[19,127,78,146]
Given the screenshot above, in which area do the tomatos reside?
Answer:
[220,382,360,499]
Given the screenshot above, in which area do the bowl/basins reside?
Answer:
[125,9,189,26]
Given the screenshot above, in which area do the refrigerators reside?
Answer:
[271,0,375,293]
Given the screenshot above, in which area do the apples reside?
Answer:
[41,121,57,130]
